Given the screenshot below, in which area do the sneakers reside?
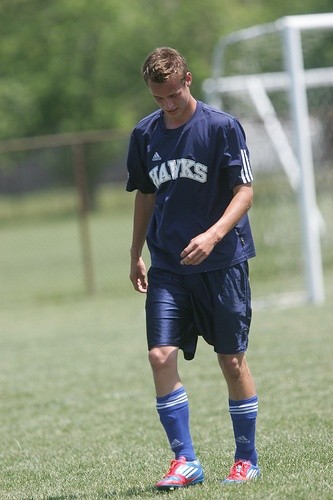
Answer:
[155,457,203,489]
[220,459,260,485]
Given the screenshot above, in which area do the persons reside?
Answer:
[124,47,264,490]
